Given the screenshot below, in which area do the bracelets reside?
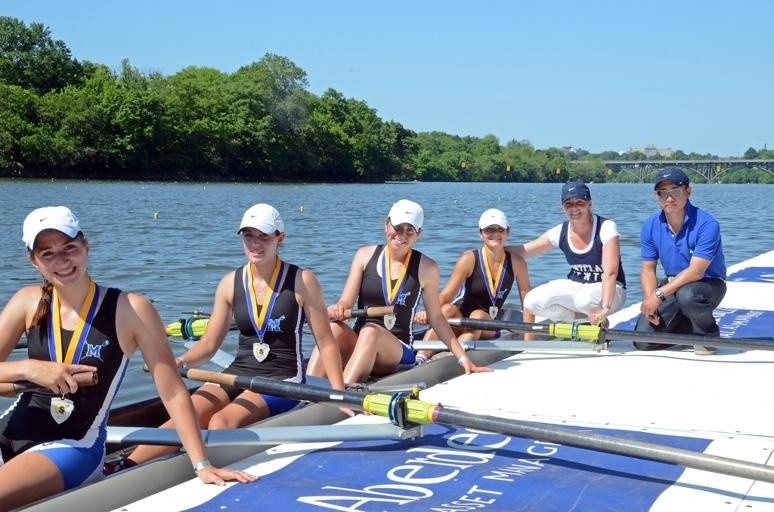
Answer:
[458,354,469,364]
[602,304,611,310]
[192,459,212,474]
[177,356,188,368]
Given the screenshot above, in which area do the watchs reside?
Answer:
[655,288,666,302]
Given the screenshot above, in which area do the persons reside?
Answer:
[0,206,257,512]
[633,167,727,355]
[524,180,626,324]
[305,199,494,384]
[114,203,375,472]
[414,209,535,365]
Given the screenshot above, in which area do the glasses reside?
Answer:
[653,184,686,201]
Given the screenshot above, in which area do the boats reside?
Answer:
[3,299,558,512]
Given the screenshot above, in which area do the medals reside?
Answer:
[49,397,74,425]
[384,314,396,331]
[489,305,498,319]
[252,342,270,363]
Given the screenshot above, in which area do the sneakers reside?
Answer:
[101,453,137,475]
[693,346,718,355]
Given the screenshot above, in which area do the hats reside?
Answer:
[560,182,591,204]
[653,168,689,191]
[478,208,510,231]
[385,199,424,233]
[236,203,284,237]
[21,205,84,251]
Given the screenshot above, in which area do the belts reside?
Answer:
[667,277,675,282]
